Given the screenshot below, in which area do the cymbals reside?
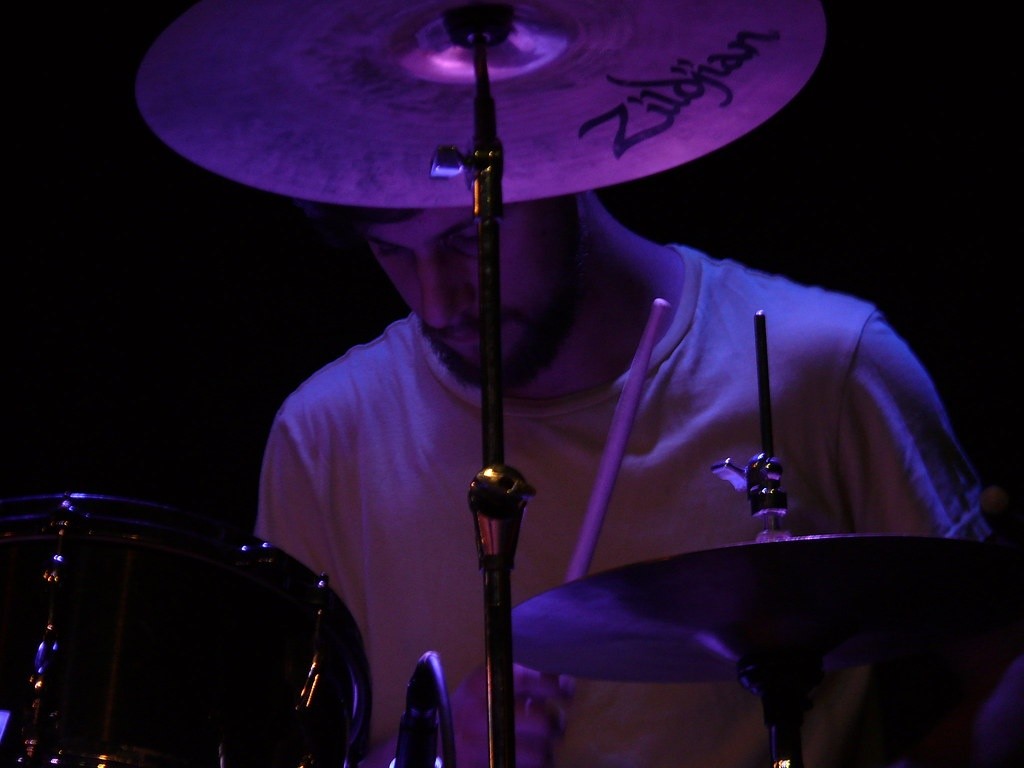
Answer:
[136,0,823,204]
[511,535,1024,684]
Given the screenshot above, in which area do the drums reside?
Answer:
[0,494,376,767]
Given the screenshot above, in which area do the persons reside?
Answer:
[254,194,991,768]
